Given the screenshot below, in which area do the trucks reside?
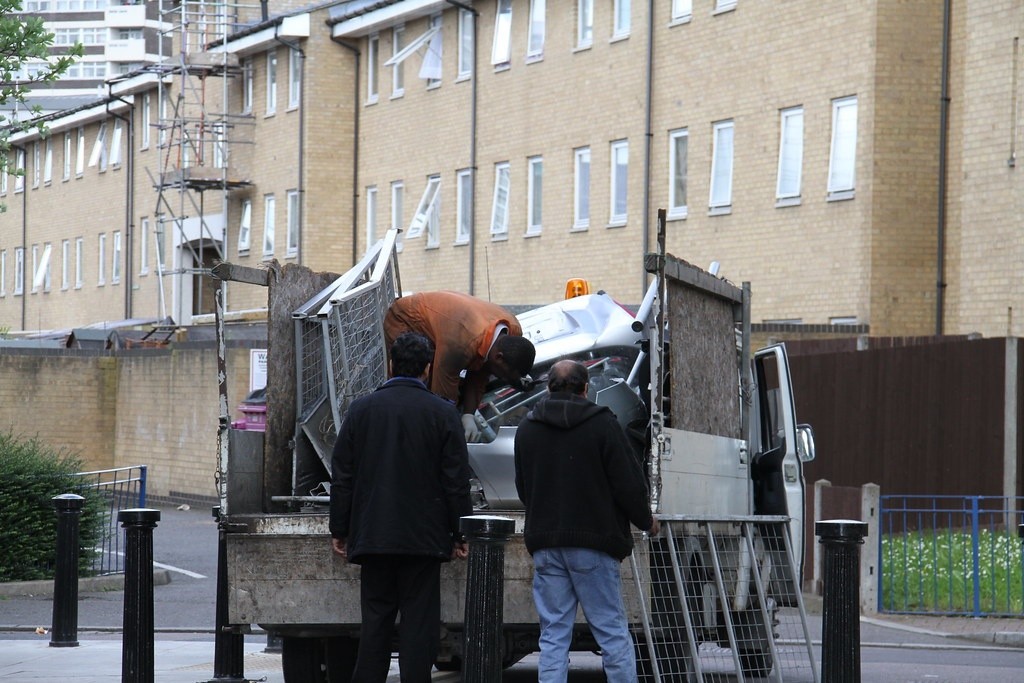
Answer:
[213,252,817,683]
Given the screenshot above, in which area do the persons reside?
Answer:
[383,290,536,442]
[513,359,662,683]
[328,333,474,683]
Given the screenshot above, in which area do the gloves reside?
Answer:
[461,414,482,443]
